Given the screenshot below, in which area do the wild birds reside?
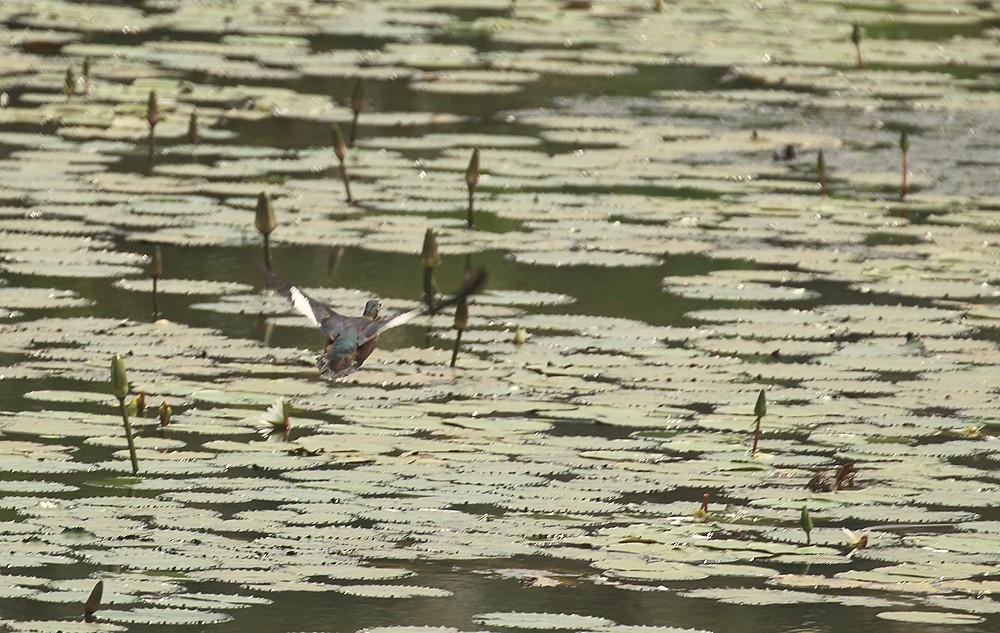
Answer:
[247,251,485,379]
